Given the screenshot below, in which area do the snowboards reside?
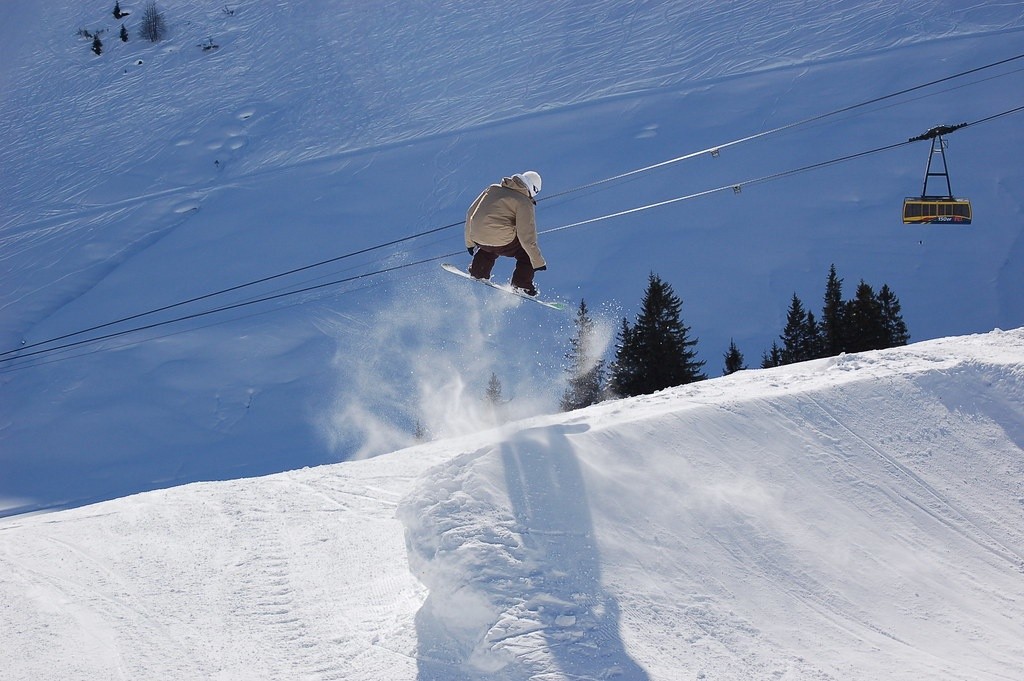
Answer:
[440,262,562,311]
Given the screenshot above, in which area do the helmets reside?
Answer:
[523,171,541,197]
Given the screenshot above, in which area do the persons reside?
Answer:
[462,170,548,299]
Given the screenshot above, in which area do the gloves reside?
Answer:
[534,266,546,271]
[468,247,474,255]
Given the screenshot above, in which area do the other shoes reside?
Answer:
[512,280,537,296]
